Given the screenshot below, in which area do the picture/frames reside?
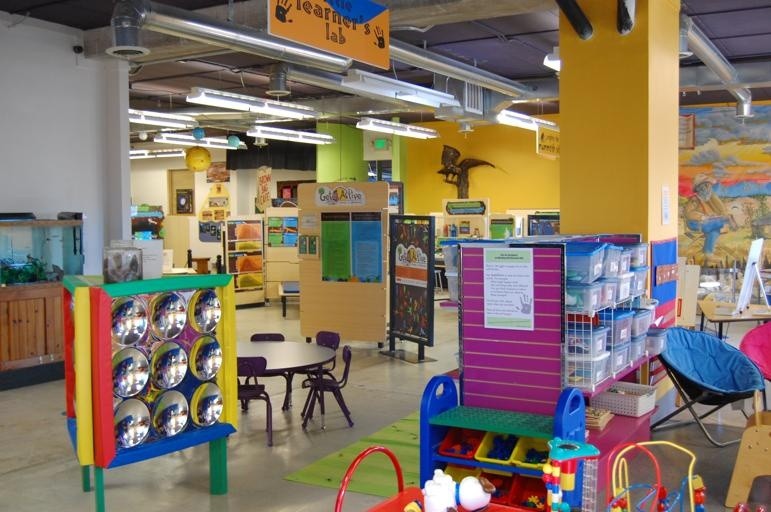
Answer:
[679,114,696,150]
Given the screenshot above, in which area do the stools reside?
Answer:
[435,270,443,291]
[278,281,300,317]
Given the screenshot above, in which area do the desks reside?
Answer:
[698,299,770,340]
[237,342,335,430]
[758,269,771,305]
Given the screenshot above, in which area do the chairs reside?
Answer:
[650,328,765,447]
[301,344,353,428]
[303,331,340,402]
[738,322,771,418]
[236,357,273,445]
[246,334,294,394]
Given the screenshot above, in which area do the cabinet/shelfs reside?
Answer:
[0,297,64,370]
[583,356,658,493]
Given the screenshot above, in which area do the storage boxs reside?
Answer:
[565,241,669,385]
[439,428,551,512]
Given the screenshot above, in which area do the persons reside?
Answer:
[684,173,738,255]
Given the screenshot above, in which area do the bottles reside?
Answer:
[449,225,457,237]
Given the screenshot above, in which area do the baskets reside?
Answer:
[589,381,658,417]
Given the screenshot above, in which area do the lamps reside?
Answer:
[153,131,248,151]
[185,85,320,121]
[340,70,455,109]
[127,148,186,161]
[355,117,440,141]
[245,124,335,146]
[495,109,556,134]
[128,108,199,130]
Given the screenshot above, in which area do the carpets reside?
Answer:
[282,411,420,497]
